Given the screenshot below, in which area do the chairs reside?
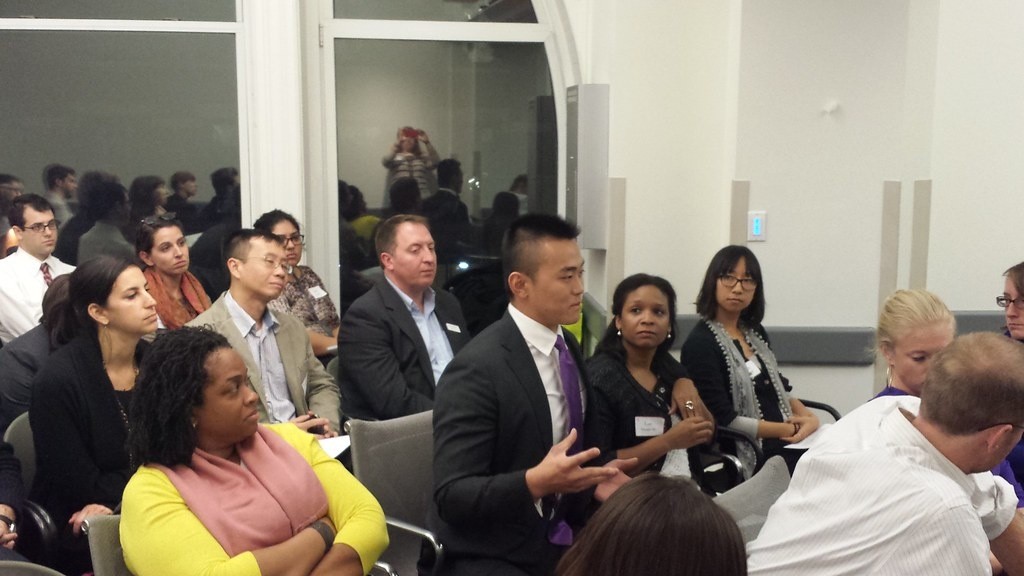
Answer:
[345,409,444,576]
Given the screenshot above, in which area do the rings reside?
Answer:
[685,401,694,410]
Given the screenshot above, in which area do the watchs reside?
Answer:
[0,515,17,533]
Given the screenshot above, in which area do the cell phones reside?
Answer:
[305,413,327,435]
[403,128,420,138]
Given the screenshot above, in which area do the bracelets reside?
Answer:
[793,423,800,436]
[310,521,335,553]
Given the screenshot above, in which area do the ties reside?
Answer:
[40,262,53,288]
[553,334,584,457]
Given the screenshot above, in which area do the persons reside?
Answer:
[867,289,1023,508]
[745,330,1024,576]
[680,245,819,497]
[0,126,639,576]
[118,327,390,576]
[996,261,1024,491]
[584,274,714,478]
[556,472,749,576]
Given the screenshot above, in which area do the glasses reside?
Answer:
[138,212,178,226]
[278,234,304,248]
[247,256,293,274]
[718,272,756,291]
[120,200,133,206]
[996,296,1024,310]
[24,218,61,232]
[2,187,23,193]
